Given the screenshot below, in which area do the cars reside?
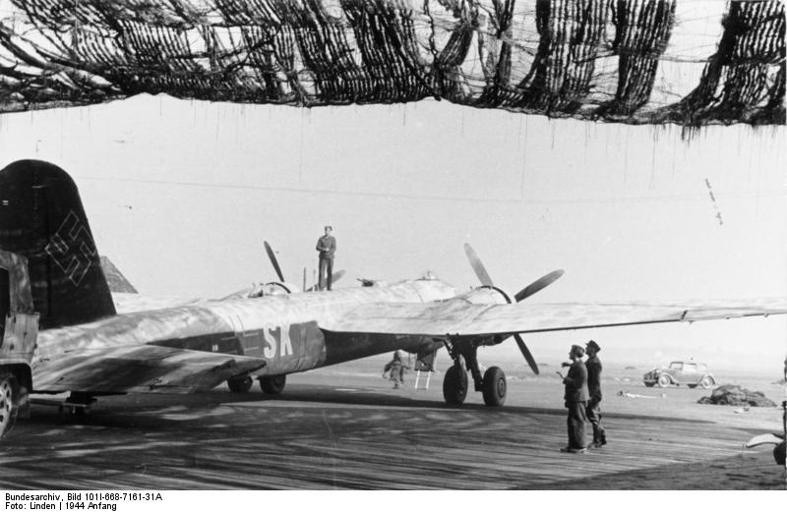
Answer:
[643,358,717,390]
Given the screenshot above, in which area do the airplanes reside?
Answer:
[0,149,787,448]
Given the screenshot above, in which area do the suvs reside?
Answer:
[0,250,41,444]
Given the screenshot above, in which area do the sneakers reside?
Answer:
[560,447,586,454]
[588,440,607,448]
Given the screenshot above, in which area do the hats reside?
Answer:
[587,340,600,353]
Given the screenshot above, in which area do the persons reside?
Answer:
[382,351,412,389]
[559,339,608,449]
[556,344,591,454]
[315,225,338,292]
